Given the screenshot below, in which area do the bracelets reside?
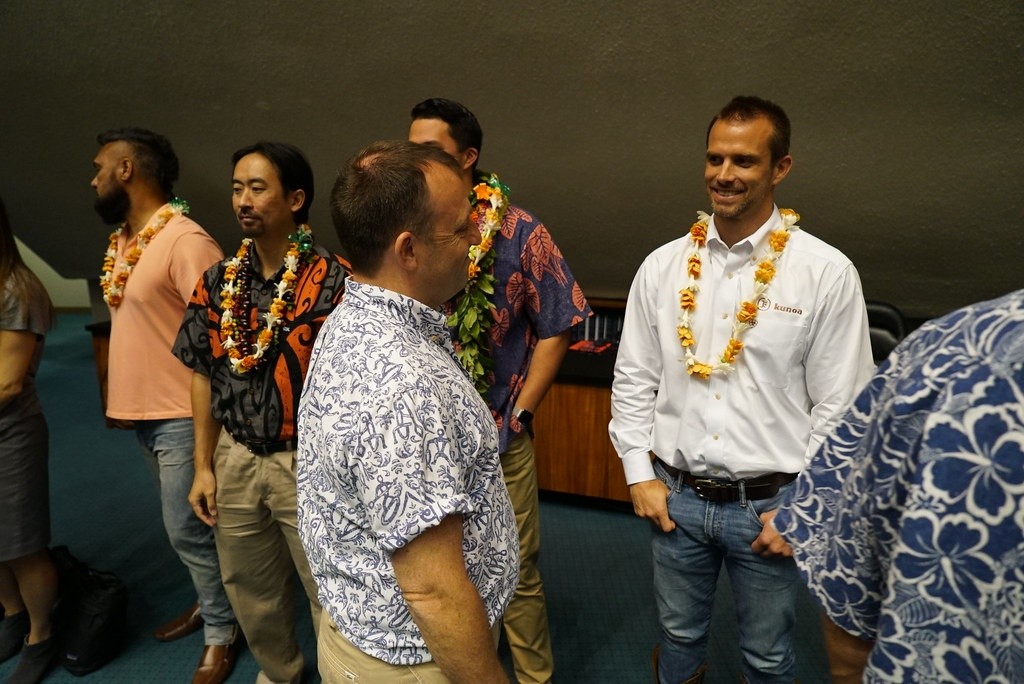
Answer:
[511,404,533,426]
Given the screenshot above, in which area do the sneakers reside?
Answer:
[45,545,89,573]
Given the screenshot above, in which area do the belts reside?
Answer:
[655,452,801,504]
[222,420,297,457]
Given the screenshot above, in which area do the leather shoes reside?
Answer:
[192,636,244,684]
[152,601,204,642]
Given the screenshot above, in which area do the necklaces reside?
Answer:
[467,173,504,281]
[99,196,189,309]
[676,204,800,384]
[223,225,311,373]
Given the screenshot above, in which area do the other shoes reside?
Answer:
[6,630,62,684]
[0,608,30,664]
[63,567,132,677]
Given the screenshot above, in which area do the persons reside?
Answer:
[0,193,61,684]
[90,97,594,684]
[770,289,1023,683]
[608,95,878,684]
